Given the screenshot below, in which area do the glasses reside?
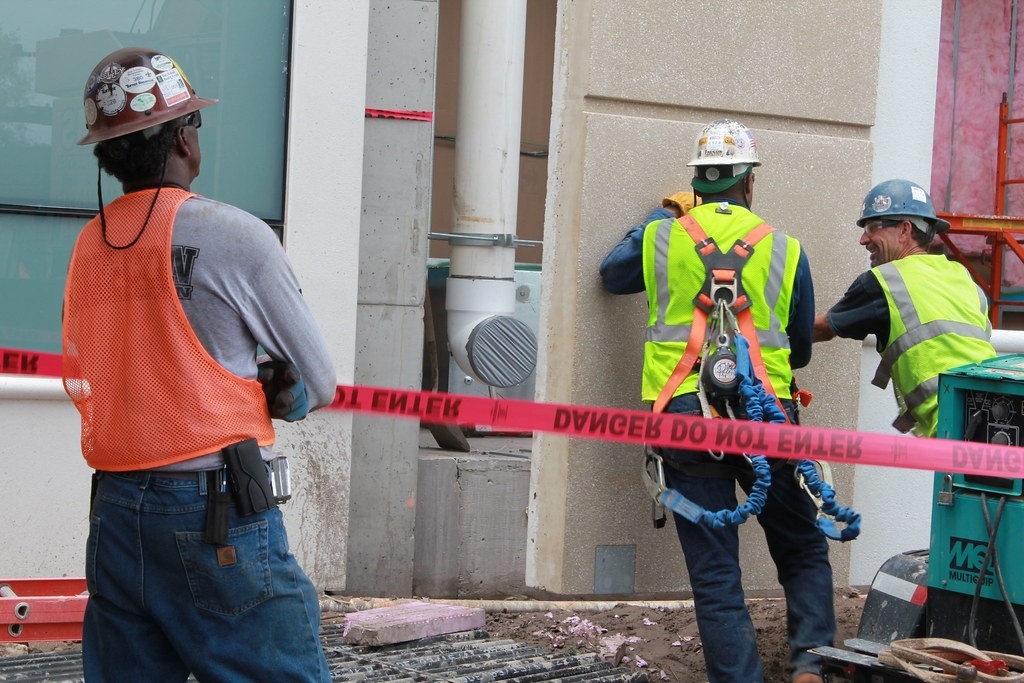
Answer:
[165,109,203,136]
[863,220,885,235]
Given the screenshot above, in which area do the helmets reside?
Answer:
[856,179,951,233]
[685,119,762,167]
[75,48,216,145]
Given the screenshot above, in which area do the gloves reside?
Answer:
[663,191,702,218]
[257,361,309,423]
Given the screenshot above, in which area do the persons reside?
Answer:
[64,45,338,683]
[601,117,835,683]
[817,179,1000,437]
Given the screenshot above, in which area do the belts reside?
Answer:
[135,471,214,483]
